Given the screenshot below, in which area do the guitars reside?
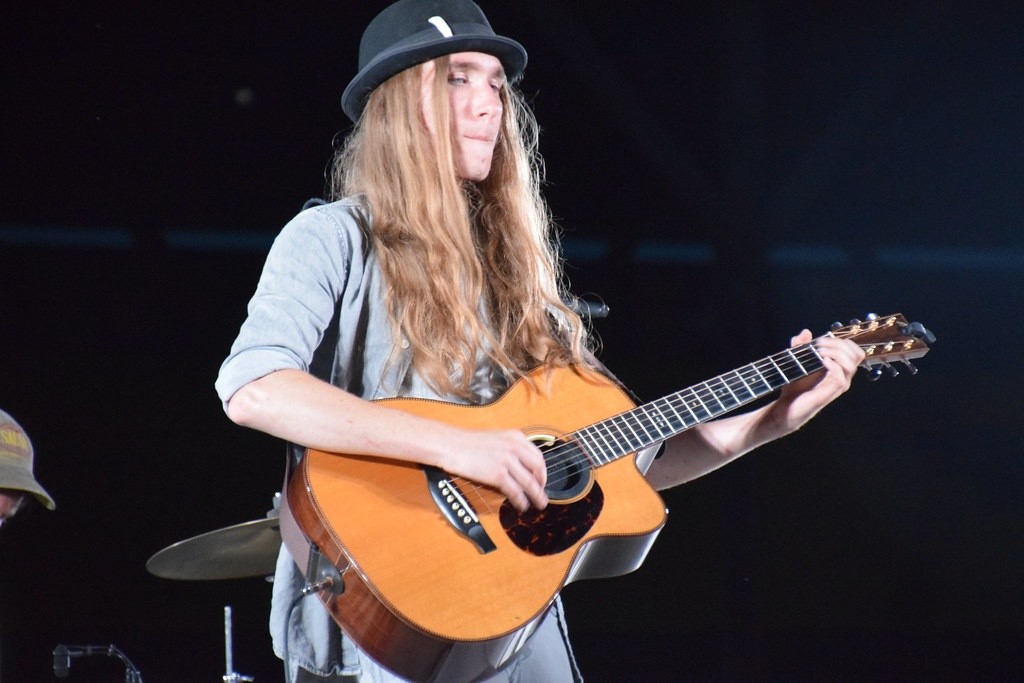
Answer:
[287,309,938,683]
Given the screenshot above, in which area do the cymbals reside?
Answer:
[143,510,282,582]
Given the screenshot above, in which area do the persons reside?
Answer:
[215,0,866,683]
[0,410,56,531]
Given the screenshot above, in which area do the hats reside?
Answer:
[339,0,528,124]
[1,409,57,511]
